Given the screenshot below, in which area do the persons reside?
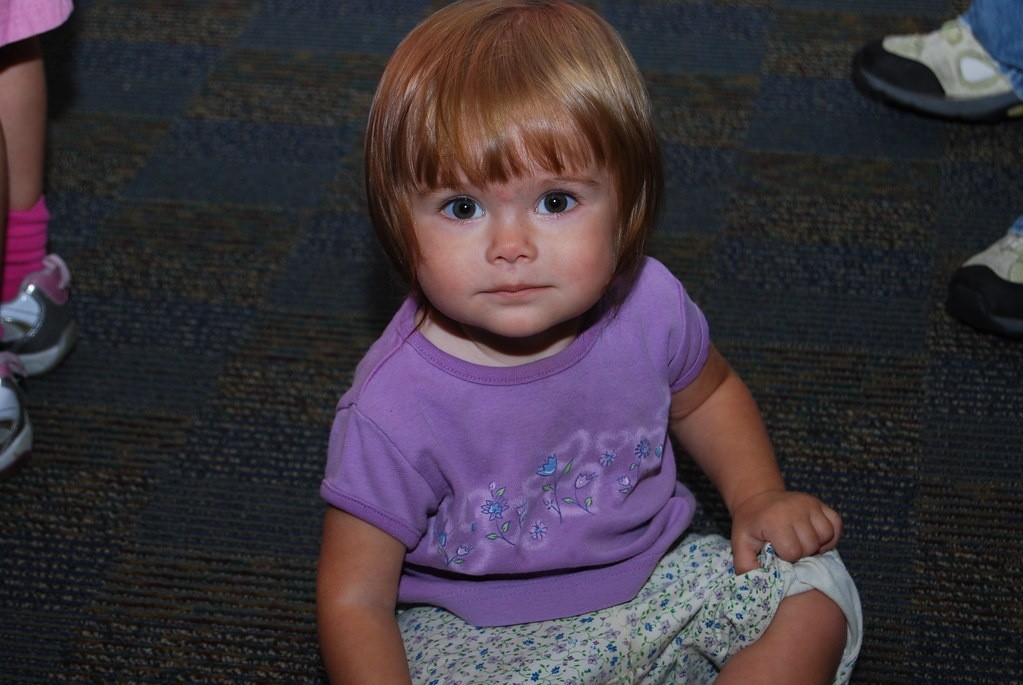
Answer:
[318,0,864,685]
[0,0,84,472]
[851,0,1023,343]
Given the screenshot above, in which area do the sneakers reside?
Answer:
[946,231,1022,339]
[855,16,1022,120]
[0,351,36,470]
[1,253,77,376]
